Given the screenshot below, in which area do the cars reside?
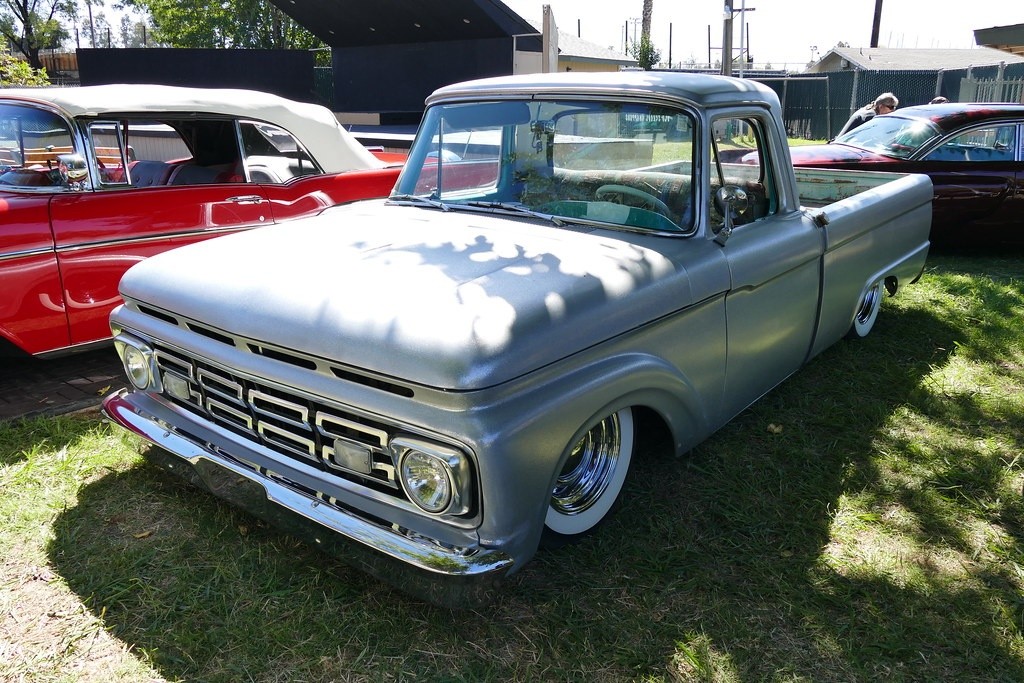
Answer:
[712,102,1024,244]
[0,84,498,375]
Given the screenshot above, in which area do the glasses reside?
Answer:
[882,103,897,111]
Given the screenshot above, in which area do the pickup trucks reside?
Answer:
[100,72,936,578]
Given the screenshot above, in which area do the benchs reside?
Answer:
[521,167,763,237]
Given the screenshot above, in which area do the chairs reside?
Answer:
[113,159,234,186]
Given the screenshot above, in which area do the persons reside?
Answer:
[836,92,899,141]
[929,96,949,104]
